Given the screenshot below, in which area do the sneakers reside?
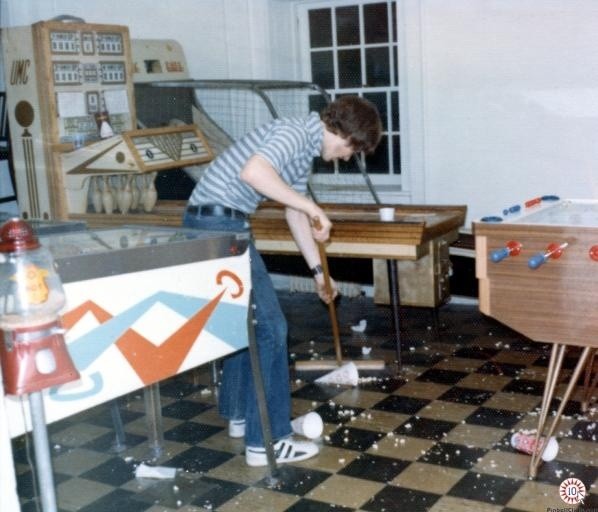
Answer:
[229,419,247,439]
[245,438,320,466]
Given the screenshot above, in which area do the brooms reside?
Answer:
[291,216,396,383]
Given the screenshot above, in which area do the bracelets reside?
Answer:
[310,264,323,275]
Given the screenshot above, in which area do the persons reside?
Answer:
[182,95,385,467]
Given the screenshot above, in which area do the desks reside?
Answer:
[66,198,468,366]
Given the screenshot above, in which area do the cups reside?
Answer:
[379,207,396,223]
[510,432,559,463]
